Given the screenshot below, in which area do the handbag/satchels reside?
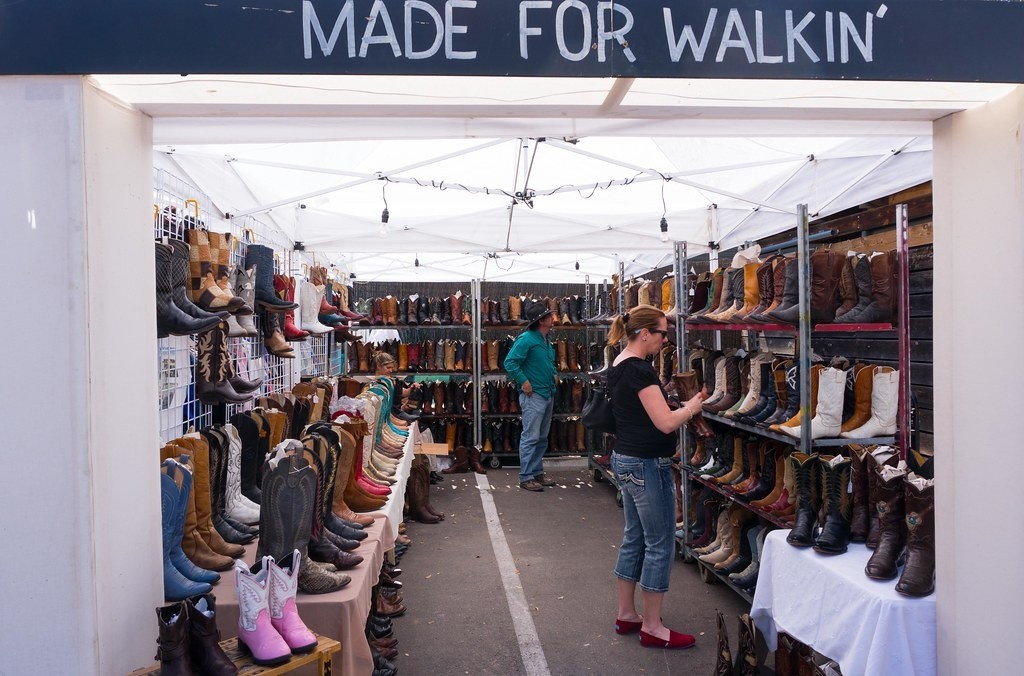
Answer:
[581,385,615,435]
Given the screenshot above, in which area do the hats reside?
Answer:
[525,302,558,329]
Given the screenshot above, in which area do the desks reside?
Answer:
[165,419,421,675]
[750,528,935,676]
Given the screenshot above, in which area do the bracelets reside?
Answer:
[685,407,693,419]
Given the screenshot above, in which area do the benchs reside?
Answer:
[123,629,342,676]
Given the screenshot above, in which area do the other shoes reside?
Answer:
[639,629,696,650]
[430,471,444,484]
[616,616,663,635]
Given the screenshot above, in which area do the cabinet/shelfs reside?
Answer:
[339,202,910,613]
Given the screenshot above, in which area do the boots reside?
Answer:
[679,245,889,591]
[714,609,759,676]
[587,288,620,324]
[350,288,485,474]
[623,271,678,317]
[787,444,935,598]
[481,378,588,415]
[652,341,679,398]
[672,368,716,439]
[481,336,587,373]
[482,416,585,453]
[155,227,421,674]
[409,455,445,523]
[481,293,586,327]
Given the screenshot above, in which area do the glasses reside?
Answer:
[634,328,667,339]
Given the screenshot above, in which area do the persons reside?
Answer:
[503,303,558,491]
[607,305,705,650]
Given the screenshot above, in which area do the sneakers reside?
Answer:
[535,475,555,485]
[520,479,544,491]
